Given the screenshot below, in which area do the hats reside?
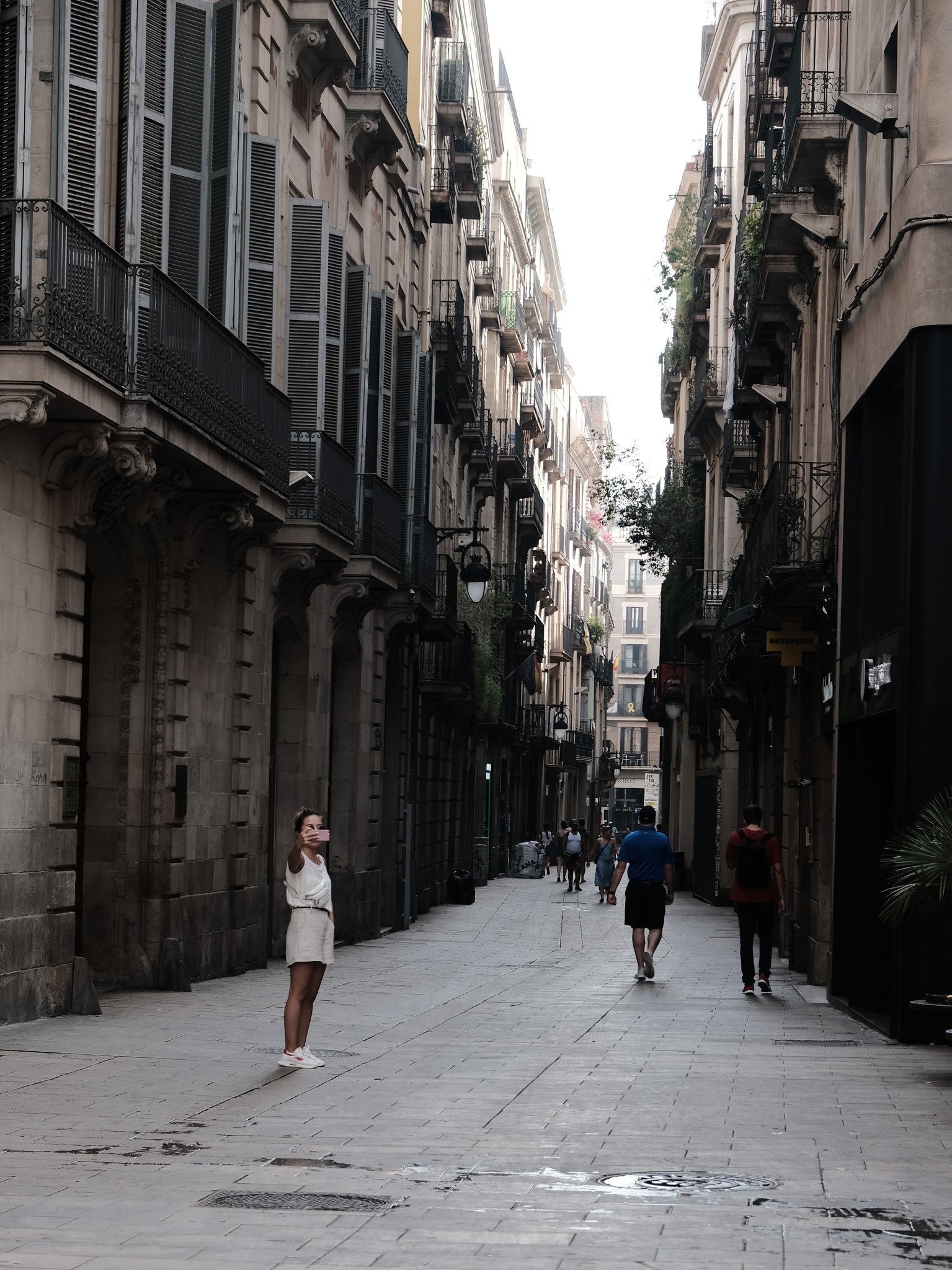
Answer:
[570,819,578,826]
[609,821,613,824]
[604,820,607,822]
[639,805,656,819]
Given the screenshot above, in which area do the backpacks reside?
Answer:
[736,828,775,888]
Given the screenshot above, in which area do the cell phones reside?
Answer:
[307,830,330,841]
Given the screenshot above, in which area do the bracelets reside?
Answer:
[608,892,616,895]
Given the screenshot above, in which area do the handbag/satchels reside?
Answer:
[594,851,600,864]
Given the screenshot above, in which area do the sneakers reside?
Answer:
[757,973,772,992]
[277,1047,325,1068]
[742,984,754,994]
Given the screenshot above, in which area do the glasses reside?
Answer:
[308,825,327,830]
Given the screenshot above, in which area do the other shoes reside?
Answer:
[606,892,608,902]
[557,878,560,882]
[634,968,645,978]
[567,886,573,891]
[643,951,655,978]
[574,878,576,882]
[599,898,604,902]
[546,867,550,875]
[563,878,567,881]
[581,880,586,883]
[575,884,582,891]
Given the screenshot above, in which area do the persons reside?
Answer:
[607,805,674,979]
[724,805,784,995]
[537,818,630,904]
[277,806,336,1068]
